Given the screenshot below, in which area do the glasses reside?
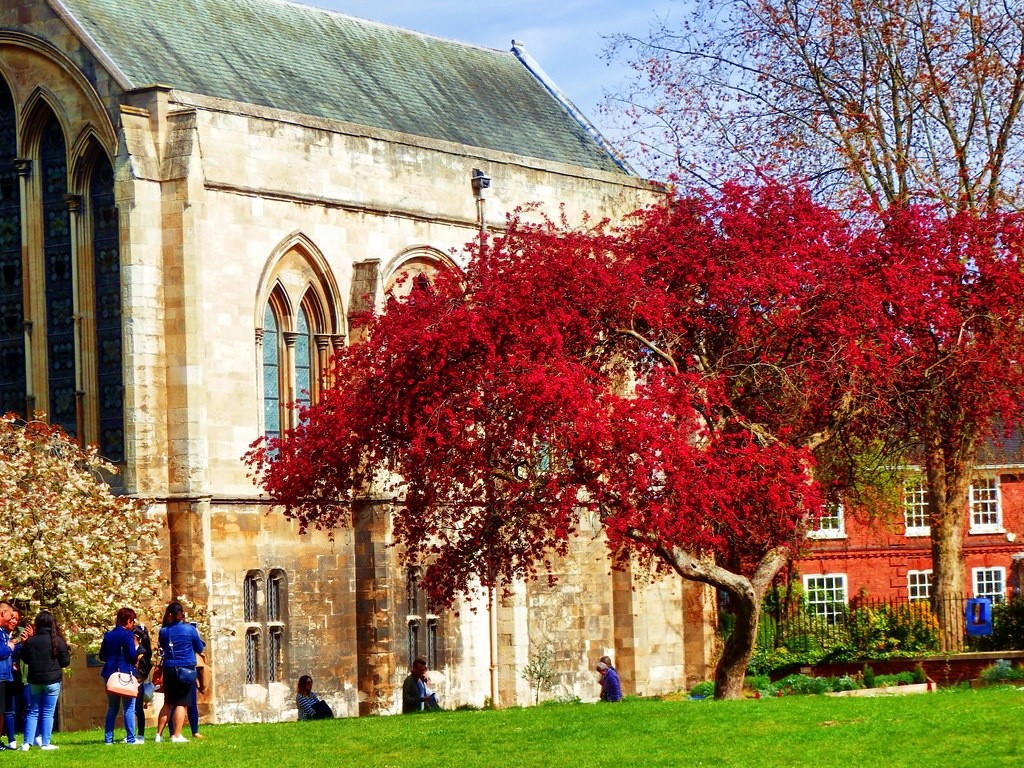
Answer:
[306,681,313,685]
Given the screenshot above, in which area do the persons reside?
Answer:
[296,674,321,721]
[168,668,206,739]
[18,611,71,751]
[597,656,623,703]
[120,624,153,745]
[98,608,143,744]
[156,602,203,745]
[402,658,436,714]
[0,601,43,750]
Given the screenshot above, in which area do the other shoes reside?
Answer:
[134,738,141,744]
[0,742,16,750]
[41,744,59,750]
[105,740,117,745]
[193,733,205,739]
[119,737,128,743]
[138,735,144,743]
[19,744,29,751]
[33,736,42,746]
[8,741,17,749]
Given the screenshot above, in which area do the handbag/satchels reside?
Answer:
[306,700,334,720]
[142,682,160,709]
[106,672,138,698]
[177,667,197,684]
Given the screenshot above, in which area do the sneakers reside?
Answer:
[154,733,165,743]
[171,734,190,742]
